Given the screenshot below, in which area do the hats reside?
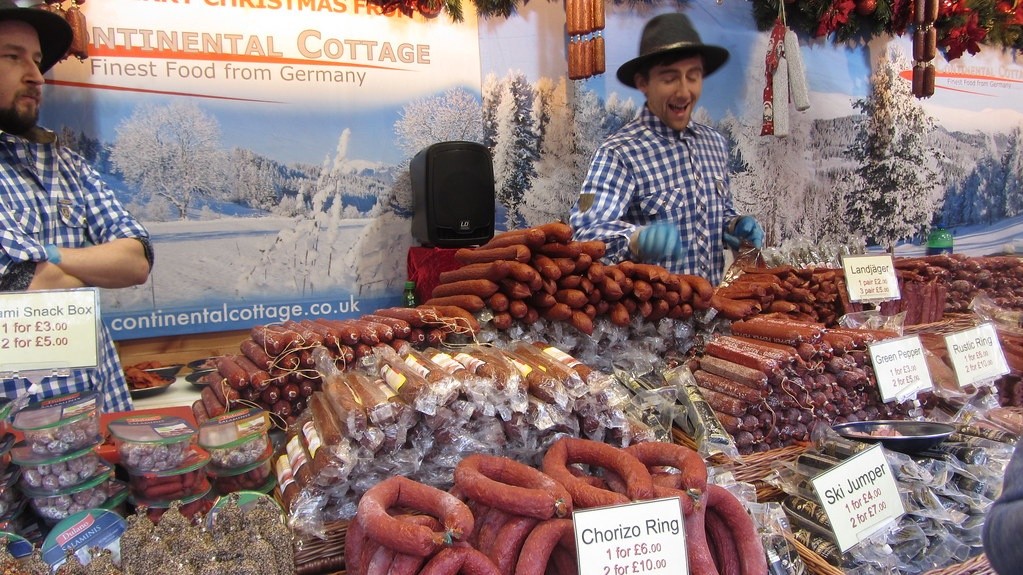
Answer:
[0,1,74,75]
[617,13,731,88]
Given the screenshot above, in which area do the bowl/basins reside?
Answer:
[829,420,956,457]
[1,391,286,572]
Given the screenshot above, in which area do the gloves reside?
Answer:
[636,223,681,264]
[722,215,764,251]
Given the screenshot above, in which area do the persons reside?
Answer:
[566,11,763,293]
[0,0,155,463]
[980,422,1023,575]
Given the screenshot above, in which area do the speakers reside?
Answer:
[410,141,494,246]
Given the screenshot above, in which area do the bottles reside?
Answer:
[400,280,420,308]
[926,223,953,258]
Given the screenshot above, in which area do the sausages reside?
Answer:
[567,0,605,83]
[911,0,940,102]
[0,224,1023,575]
